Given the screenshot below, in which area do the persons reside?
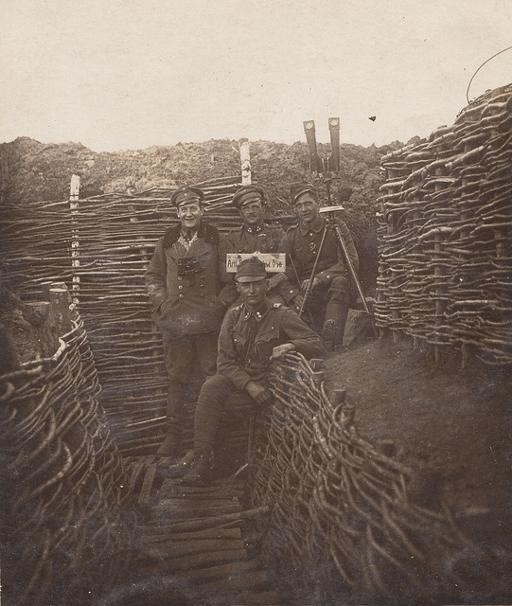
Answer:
[215,184,286,276]
[275,183,362,348]
[144,186,242,470]
[166,260,325,487]
[334,187,380,297]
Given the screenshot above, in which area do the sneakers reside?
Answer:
[181,453,211,490]
[320,318,341,353]
[167,448,194,479]
[208,453,228,482]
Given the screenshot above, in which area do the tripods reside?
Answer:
[297,181,379,339]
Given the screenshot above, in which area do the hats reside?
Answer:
[289,181,318,204]
[230,183,271,210]
[170,184,206,209]
[233,256,270,284]
